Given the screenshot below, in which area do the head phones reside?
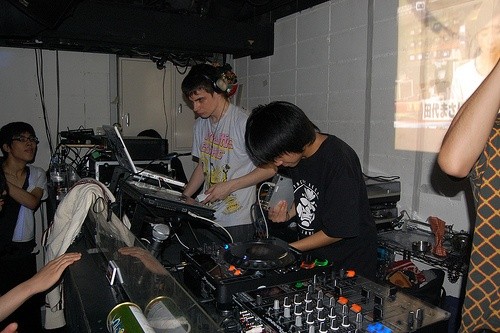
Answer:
[191,67,227,93]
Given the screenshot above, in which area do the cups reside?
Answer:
[50,172,65,191]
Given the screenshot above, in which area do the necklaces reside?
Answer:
[208,101,226,204]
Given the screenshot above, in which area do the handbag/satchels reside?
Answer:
[399,269,446,306]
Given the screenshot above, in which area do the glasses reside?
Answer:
[12,136,39,143]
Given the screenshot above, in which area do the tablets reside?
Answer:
[267,176,295,212]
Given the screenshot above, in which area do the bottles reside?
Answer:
[49,155,59,190]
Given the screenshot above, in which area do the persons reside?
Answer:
[449,0,500,101]
[438,58,500,333]
[0,252,81,333]
[182,64,275,256]
[0,122,42,333]
[245,101,379,281]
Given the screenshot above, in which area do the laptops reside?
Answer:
[102,125,185,187]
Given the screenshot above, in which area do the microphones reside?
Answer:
[138,224,170,284]
